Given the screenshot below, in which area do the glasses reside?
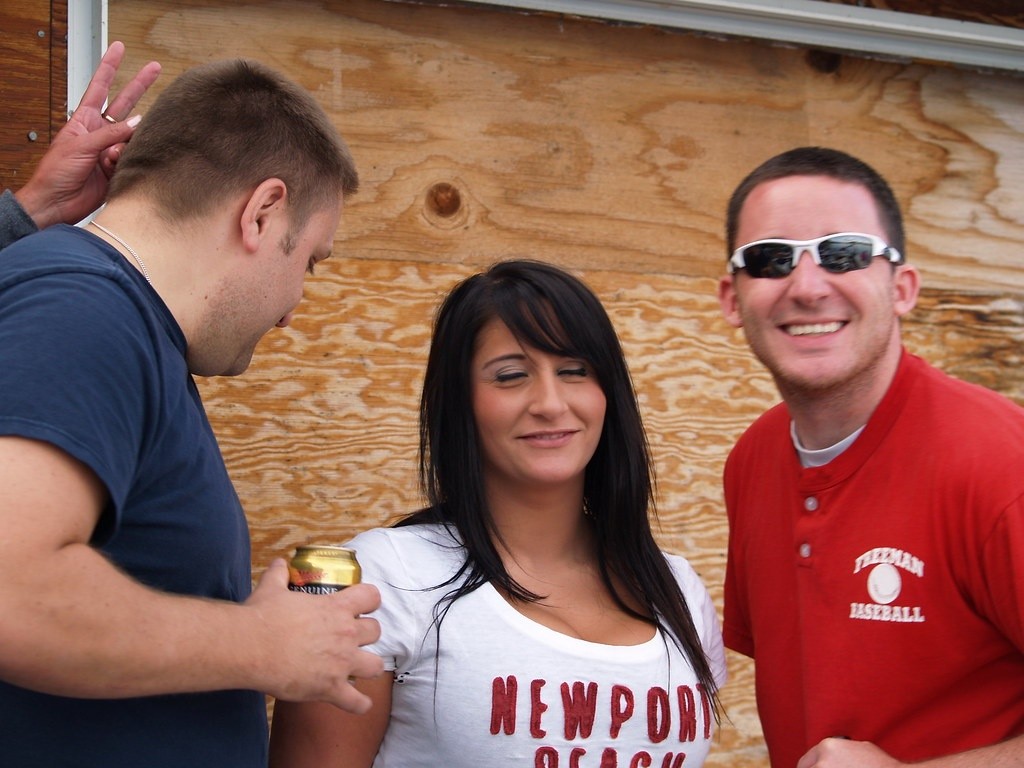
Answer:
[726,232,906,279]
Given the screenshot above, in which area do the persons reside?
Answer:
[267,258,726,768]
[0,41,384,768]
[715,146,1024,768]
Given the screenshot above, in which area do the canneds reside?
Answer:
[287,543,362,686]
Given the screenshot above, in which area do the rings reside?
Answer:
[102,112,118,124]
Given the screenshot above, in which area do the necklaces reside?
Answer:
[90,220,152,286]
